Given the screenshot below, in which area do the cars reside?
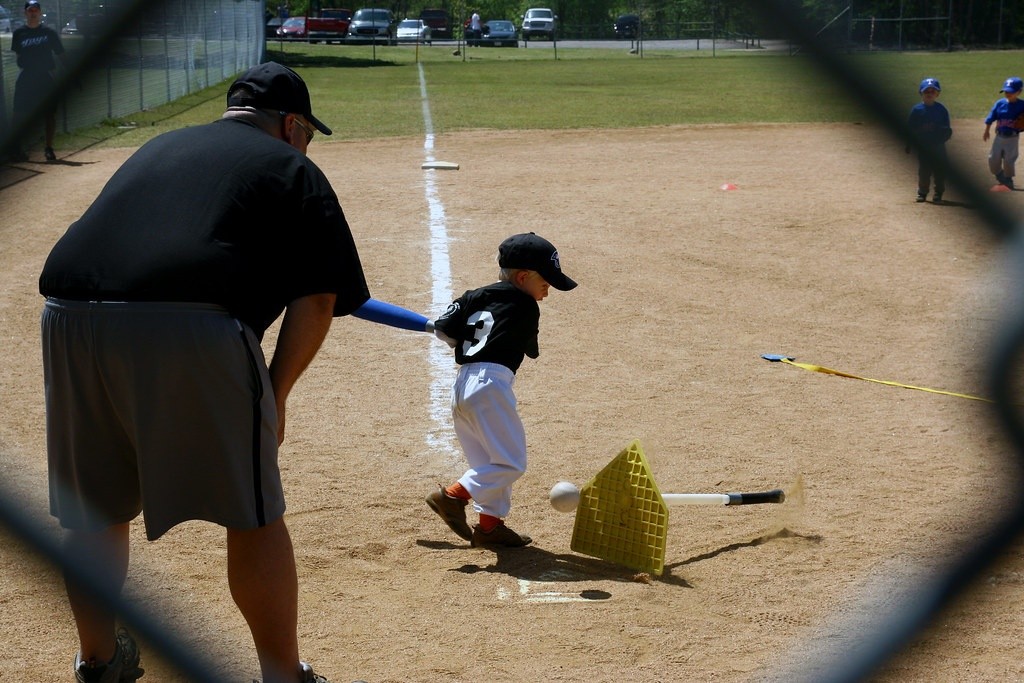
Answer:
[276,16,307,41]
[480,20,518,47]
[613,14,644,39]
[397,18,433,43]
[346,8,399,45]
[0,4,78,35]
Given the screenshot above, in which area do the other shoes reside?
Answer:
[932,193,942,202]
[73,628,140,683]
[997,170,1004,185]
[470,523,533,548]
[1004,178,1014,191]
[424,483,475,542]
[252,663,328,683]
[916,193,925,202]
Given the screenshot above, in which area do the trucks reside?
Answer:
[418,9,452,39]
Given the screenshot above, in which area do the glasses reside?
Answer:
[278,111,315,147]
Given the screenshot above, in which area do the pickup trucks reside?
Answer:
[519,8,558,41]
[305,8,354,44]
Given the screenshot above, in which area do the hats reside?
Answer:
[919,78,941,95]
[498,232,580,291]
[225,59,331,137]
[999,77,1024,93]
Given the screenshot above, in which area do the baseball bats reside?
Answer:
[349,299,435,334]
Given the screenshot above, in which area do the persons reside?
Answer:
[424,232,577,549]
[905,78,953,201]
[40,61,371,683]
[12,1,65,162]
[983,78,1024,189]
[472,10,485,47]
